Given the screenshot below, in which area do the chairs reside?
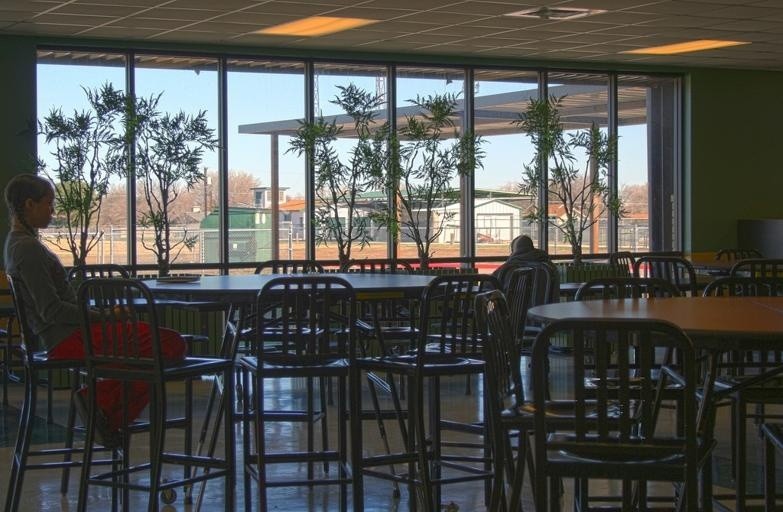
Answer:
[1,251,782,511]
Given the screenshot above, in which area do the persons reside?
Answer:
[3,173,188,450]
[480,235,560,345]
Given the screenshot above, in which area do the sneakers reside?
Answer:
[73,389,131,450]
